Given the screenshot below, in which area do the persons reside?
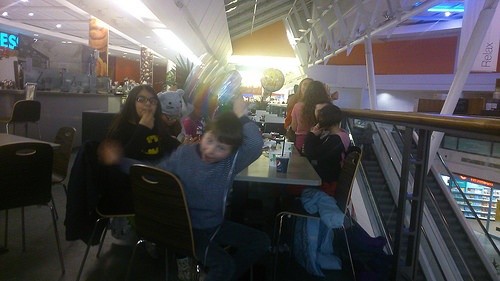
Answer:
[96,84,183,162]
[96,96,271,281]
[268,77,350,254]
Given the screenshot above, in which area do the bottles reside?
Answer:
[195,122,203,140]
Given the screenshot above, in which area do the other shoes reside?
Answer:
[269,243,290,253]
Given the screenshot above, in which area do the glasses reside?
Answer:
[136,96,159,105]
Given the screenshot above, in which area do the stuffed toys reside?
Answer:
[157,89,185,114]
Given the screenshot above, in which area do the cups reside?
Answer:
[276,156,289,173]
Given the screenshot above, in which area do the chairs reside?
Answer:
[130,163,254,281]
[0,143,65,275]
[6,101,42,140]
[52,126,75,220]
[272,146,363,281]
[76,141,171,281]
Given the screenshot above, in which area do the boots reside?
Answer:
[176,256,201,281]
[145,241,162,260]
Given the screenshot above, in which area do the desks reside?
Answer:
[0,133,62,253]
[228,136,323,256]
[250,109,286,124]
